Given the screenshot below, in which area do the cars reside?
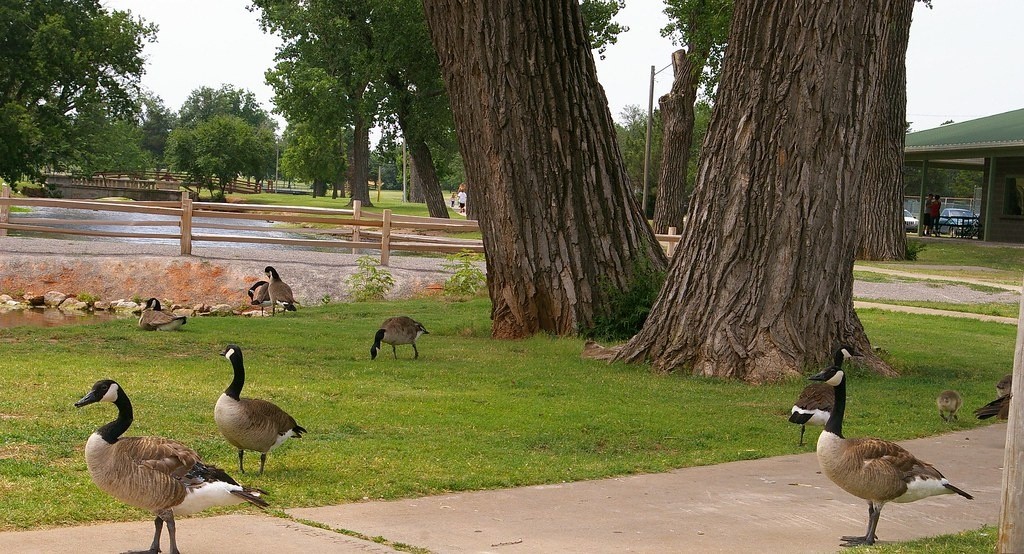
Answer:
[904,209,919,233]
[933,207,979,237]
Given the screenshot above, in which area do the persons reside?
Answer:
[451,189,467,214]
[923,194,932,237]
[928,195,942,237]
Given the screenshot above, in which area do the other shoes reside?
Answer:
[460,210,463,213]
[462,208,465,213]
[922,231,927,236]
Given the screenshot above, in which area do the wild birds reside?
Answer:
[133,297,186,332]
[370,317,428,361]
[247,264,299,316]
[787,346,975,544]
[214,344,308,472]
[73,379,271,554]
